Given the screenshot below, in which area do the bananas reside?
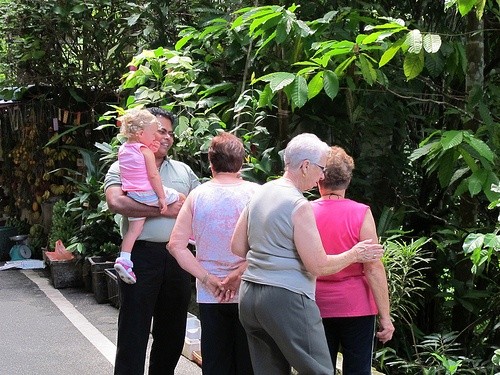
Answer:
[41,146,72,200]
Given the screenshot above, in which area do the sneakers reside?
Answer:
[189,235,195,244]
[114,258,136,284]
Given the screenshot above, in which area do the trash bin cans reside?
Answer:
[88,256,115,304]
[0,226,17,263]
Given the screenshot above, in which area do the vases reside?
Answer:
[44,251,83,288]
[0,226,17,261]
[102,268,124,309]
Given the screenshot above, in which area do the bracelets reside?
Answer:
[202,273,209,284]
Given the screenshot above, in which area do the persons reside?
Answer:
[309,145,394,375]
[231,133,385,375]
[166,134,262,375]
[104,106,201,375]
[114,108,196,285]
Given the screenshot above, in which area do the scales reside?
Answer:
[9,234,31,260]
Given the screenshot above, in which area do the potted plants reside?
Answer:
[37,201,115,303]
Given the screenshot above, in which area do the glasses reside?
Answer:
[314,163,328,173]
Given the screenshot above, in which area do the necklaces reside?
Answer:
[321,193,344,199]
